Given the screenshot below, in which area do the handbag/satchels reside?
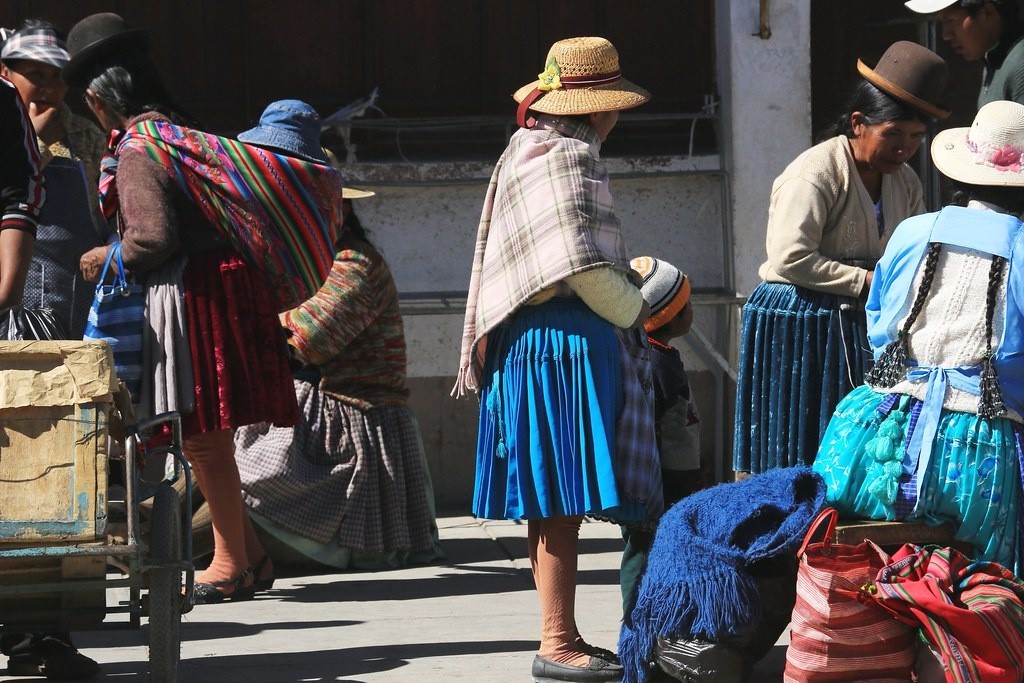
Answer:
[780,508,917,682]
[862,542,1024,683]
[83,241,147,407]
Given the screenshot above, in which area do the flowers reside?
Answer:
[991,144,1021,173]
[538,56,562,91]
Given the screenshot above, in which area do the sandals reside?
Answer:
[253,554,276,590]
[189,567,255,604]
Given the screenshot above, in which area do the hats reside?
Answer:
[322,149,376,198]
[930,100,1024,187]
[66,14,149,80]
[858,41,954,120]
[237,99,327,164]
[904,0,960,14]
[513,37,651,129]
[631,257,692,332]
[0,28,70,66]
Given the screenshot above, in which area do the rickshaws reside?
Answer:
[0,337,203,683]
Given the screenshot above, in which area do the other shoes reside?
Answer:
[7,649,101,677]
[531,645,623,683]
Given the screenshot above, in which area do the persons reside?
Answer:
[0,18,71,308]
[450,37,703,683]
[64,12,302,605]
[106,98,446,575]
[732,0,1024,581]
